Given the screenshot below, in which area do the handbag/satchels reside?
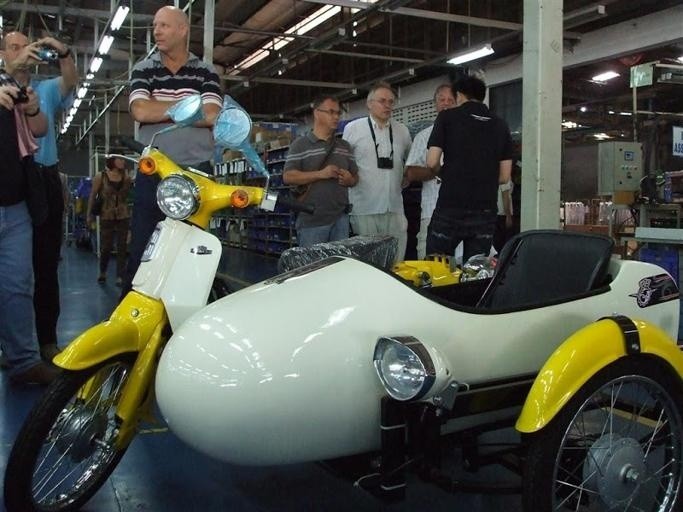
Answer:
[92,193,104,216]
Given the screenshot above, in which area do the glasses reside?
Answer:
[316,107,342,114]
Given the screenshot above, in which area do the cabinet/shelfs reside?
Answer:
[208,121,303,257]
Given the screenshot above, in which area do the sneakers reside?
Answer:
[15,363,56,384]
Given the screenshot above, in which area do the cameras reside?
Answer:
[378,158,393,168]
[37,46,59,61]
[7,87,29,105]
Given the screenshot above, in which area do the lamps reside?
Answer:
[442,0,495,66]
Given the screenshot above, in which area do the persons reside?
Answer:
[0,31,80,386]
[341,84,413,261]
[86,149,133,287]
[405,77,515,270]
[284,83,359,277]
[120,6,221,387]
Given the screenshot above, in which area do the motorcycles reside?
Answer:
[1,95,683,511]
